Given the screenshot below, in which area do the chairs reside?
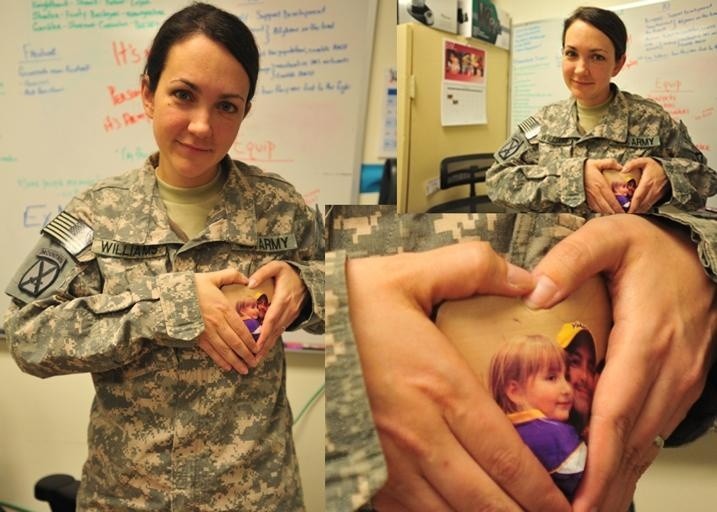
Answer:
[376,159,397,206]
[430,154,504,214]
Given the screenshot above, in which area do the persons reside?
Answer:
[235,291,272,337]
[609,176,639,209]
[325,206,716,512]
[446,49,484,78]
[488,318,598,475]
[1,2,325,511]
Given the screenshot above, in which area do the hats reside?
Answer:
[626,176,636,185]
[256,292,268,303]
[556,321,598,367]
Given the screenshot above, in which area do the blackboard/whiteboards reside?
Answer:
[1,0,379,323]
